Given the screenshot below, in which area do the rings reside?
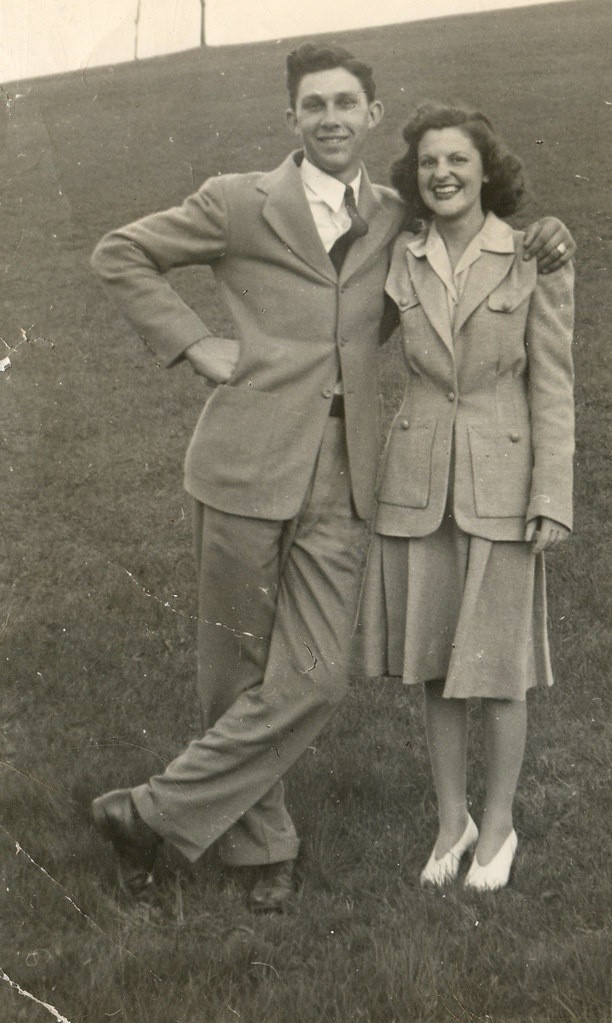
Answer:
[557,244,566,252]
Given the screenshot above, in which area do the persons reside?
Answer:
[91,42,575,909]
[362,102,575,891]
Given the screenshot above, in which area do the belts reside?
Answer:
[329,394,345,416]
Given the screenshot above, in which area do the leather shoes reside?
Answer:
[91,789,169,915]
[248,858,295,914]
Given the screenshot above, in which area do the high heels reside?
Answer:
[465,827,519,893]
[420,813,480,889]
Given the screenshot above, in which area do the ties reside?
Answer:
[327,185,369,272]
